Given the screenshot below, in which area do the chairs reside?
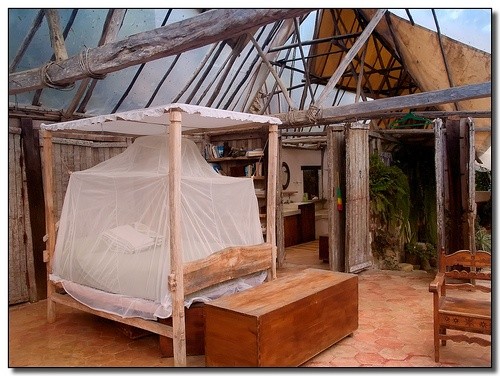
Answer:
[428,247,491,362]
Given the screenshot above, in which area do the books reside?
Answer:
[209,163,224,175]
[245,162,262,177]
[246,148,265,157]
[205,144,223,159]
[253,183,266,196]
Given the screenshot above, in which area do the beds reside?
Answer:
[39,104,283,367]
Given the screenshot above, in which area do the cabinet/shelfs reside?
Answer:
[205,125,283,268]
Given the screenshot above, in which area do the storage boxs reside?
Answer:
[158,304,203,357]
